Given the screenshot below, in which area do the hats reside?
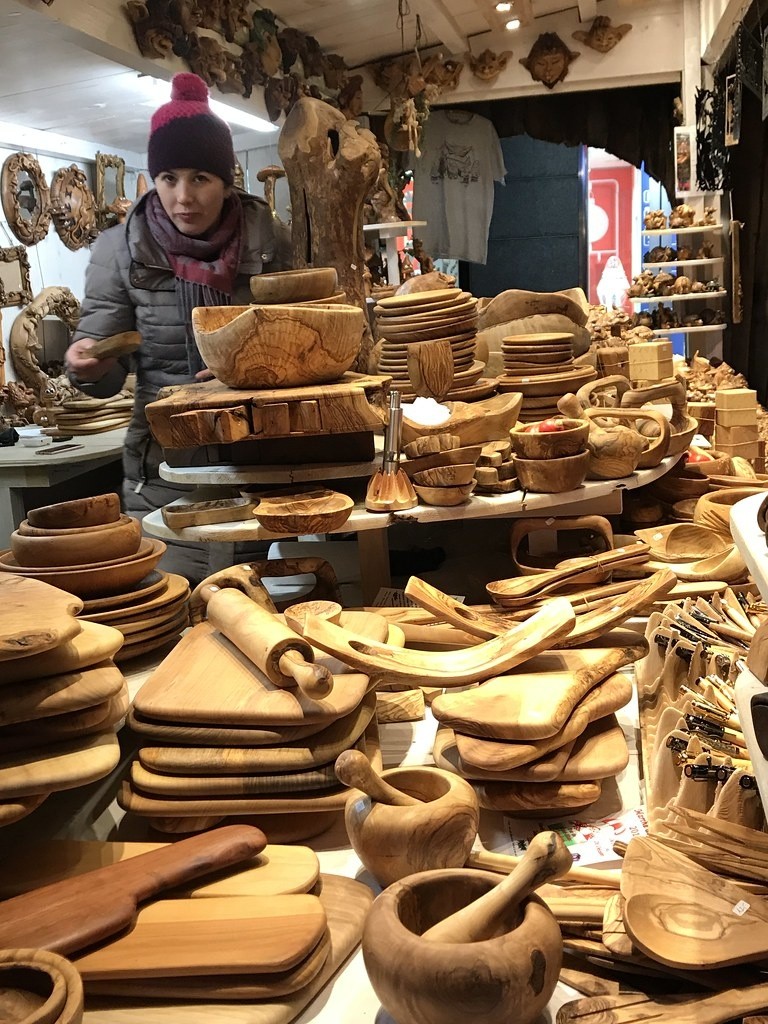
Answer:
[148,72,236,187]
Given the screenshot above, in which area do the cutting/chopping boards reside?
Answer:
[116,610,406,844]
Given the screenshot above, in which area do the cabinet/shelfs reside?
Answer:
[628,224,728,335]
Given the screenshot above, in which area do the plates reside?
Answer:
[76,568,193,663]
[373,289,598,423]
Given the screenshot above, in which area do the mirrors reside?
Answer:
[96,152,127,231]
[0,245,35,308]
[10,286,83,396]
[0,152,53,246]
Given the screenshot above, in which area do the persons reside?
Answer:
[64,72,294,591]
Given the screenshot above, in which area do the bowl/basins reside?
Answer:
[508,418,592,491]
[249,267,346,308]
[399,446,482,507]
[0,493,169,599]
